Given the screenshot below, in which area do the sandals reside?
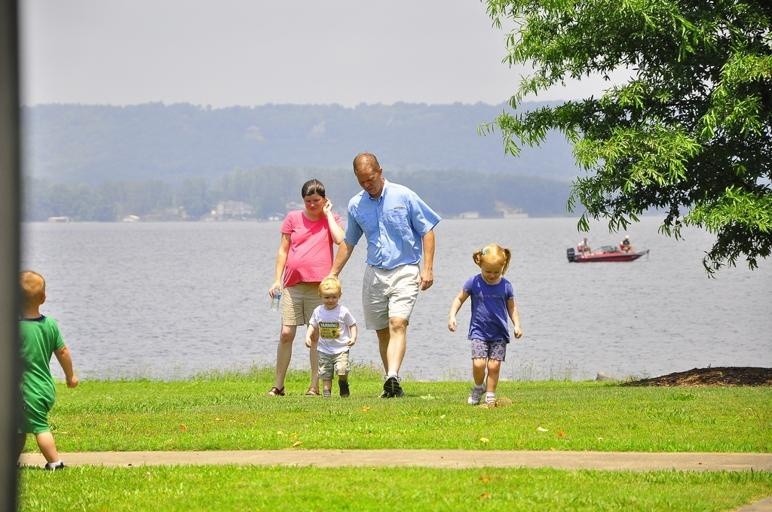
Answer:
[306,387,319,396]
[268,387,284,396]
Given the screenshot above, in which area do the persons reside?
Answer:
[306,274,357,398]
[448,244,522,405]
[329,153,443,398]
[620,235,635,254]
[578,237,590,255]
[267,178,340,398]
[15,269,79,469]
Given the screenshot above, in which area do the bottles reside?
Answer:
[269,288,281,313]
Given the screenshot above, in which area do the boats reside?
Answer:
[566,247,650,263]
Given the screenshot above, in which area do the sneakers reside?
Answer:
[44,463,64,470]
[468,385,486,404]
[338,380,349,396]
[485,393,495,405]
[383,377,403,398]
[323,389,330,397]
